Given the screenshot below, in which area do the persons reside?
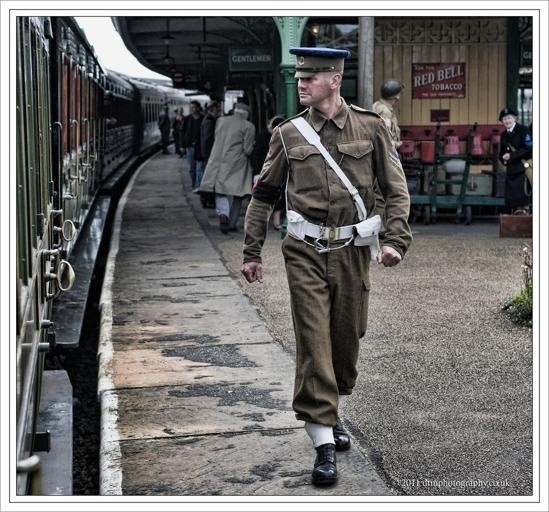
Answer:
[499,106,533,216]
[241,49,414,485]
[373,79,404,241]
[155,95,284,233]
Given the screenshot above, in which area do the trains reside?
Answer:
[17,17,210,495]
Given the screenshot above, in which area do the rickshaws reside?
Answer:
[421,120,477,225]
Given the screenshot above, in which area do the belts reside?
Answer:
[306,222,358,242]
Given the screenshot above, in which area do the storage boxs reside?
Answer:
[404,165,506,198]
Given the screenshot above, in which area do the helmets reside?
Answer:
[380,79,406,99]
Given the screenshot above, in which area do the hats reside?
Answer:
[498,108,518,121]
[235,101,253,113]
[287,44,353,80]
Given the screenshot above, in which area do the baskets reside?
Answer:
[499,208,532,239]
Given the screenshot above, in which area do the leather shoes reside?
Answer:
[333,415,351,451]
[218,212,240,235]
[311,442,339,488]
